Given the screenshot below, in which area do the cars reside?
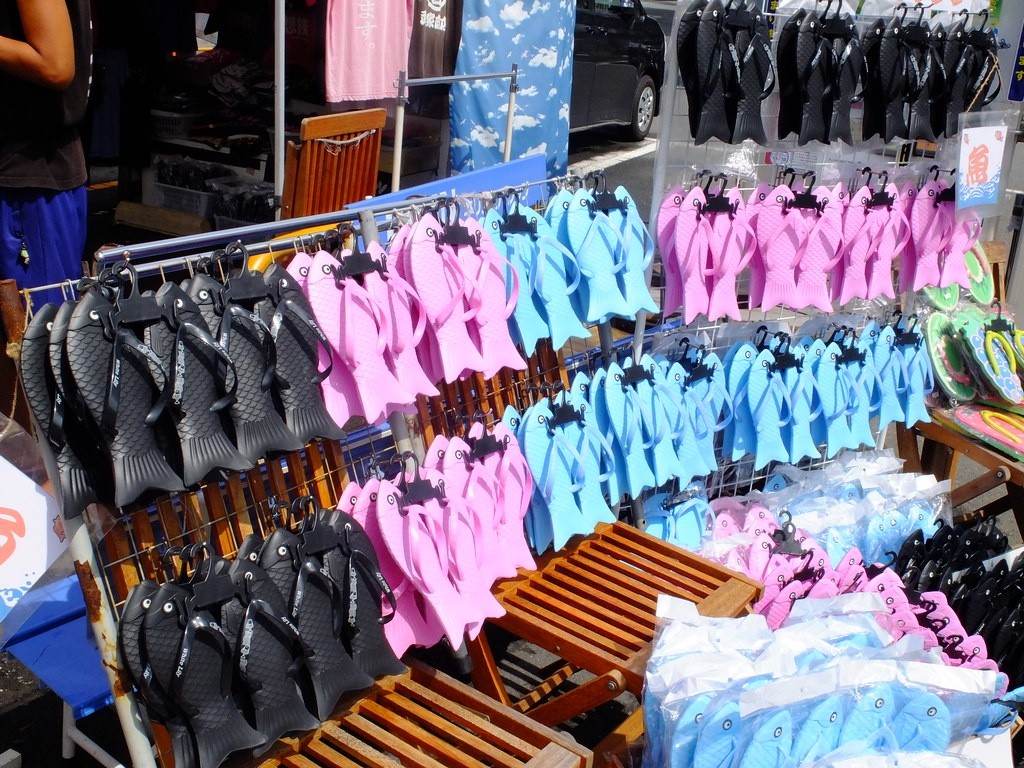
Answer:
[571,0,665,145]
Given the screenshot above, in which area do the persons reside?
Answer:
[0,0,89,317]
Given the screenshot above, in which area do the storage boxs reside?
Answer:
[152,181,217,220]
[213,213,274,231]
[151,108,205,138]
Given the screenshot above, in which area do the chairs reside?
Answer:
[893,240,1024,533]
[108,439,595,767]
[415,338,764,767]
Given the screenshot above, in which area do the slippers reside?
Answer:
[15,0,1024,768]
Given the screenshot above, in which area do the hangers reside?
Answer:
[726,0,994,48]
[55,163,1024,768]
